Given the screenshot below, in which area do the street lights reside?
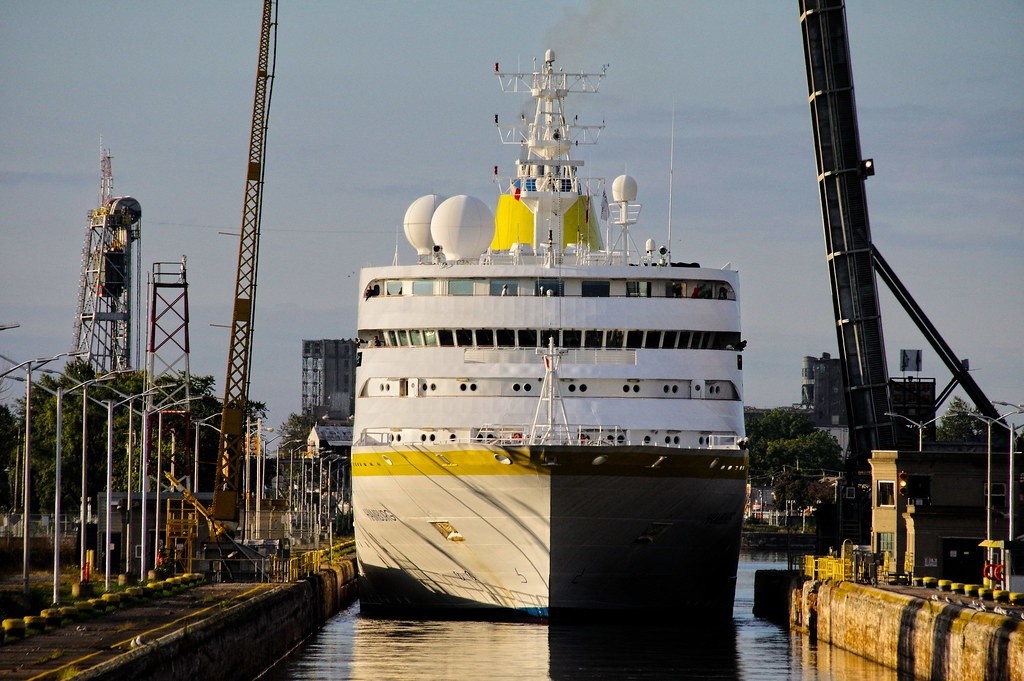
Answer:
[194,412,223,493]
[101,400,190,581]
[948,408,1024,539]
[884,413,959,452]
[64,389,159,591]
[276,440,302,500]
[262,435,292,499]
[0,358,60,378]
[36,368,136,583]
[3,376,117,604]
[0,350,89,591]
[92,384,178,573]
[120,396,203,570]
[250,428,274,436]
[967,413,1024,541]
[290,444,348,538]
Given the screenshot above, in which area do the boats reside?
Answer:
[350,48,751,624]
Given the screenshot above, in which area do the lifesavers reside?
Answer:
[984,564,994,580]
[993,565,1004,581]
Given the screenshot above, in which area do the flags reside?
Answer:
[586,190,590,223]
[601,188,610,221]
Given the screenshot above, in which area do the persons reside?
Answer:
[367,286,373,296]
[539,286,554,296]
[500,284,508,296]
[586,331,623,349]
[355,337,365,348]
[727,340,747,352]
[374,336,381,346]
[690,284,700,298]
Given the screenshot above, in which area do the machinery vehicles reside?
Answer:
[163,1,278,573]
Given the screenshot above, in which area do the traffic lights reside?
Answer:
[252,438,259,455]
[898,472,908,496]
[245,435,248,456]
